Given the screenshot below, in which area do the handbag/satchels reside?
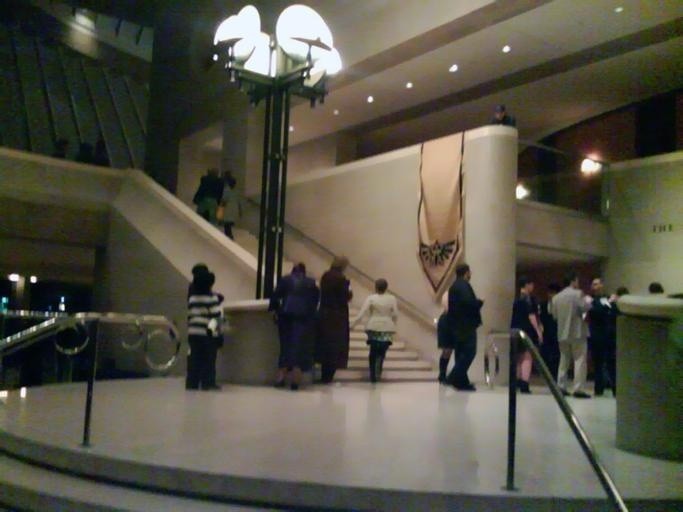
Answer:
[216,201,225,221]
[207,318,223,348]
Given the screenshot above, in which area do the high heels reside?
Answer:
[517,378,532,394]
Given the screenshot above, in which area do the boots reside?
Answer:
[438,356,450,384]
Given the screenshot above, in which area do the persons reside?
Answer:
[649,282,664,293]
[185,253,401,391]
[489,103,516,128]
[436,263,629,400]
[75,143,96,164]
[92,139,109,166]
[192,166,245,238]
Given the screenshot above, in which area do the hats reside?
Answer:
[494,104,506,112]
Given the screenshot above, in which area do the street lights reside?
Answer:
[211,2,347,300]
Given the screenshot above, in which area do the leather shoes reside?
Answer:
[573,392,592,398]
[562,390,570,396]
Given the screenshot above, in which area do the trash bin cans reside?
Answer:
[615,294,682,461]
